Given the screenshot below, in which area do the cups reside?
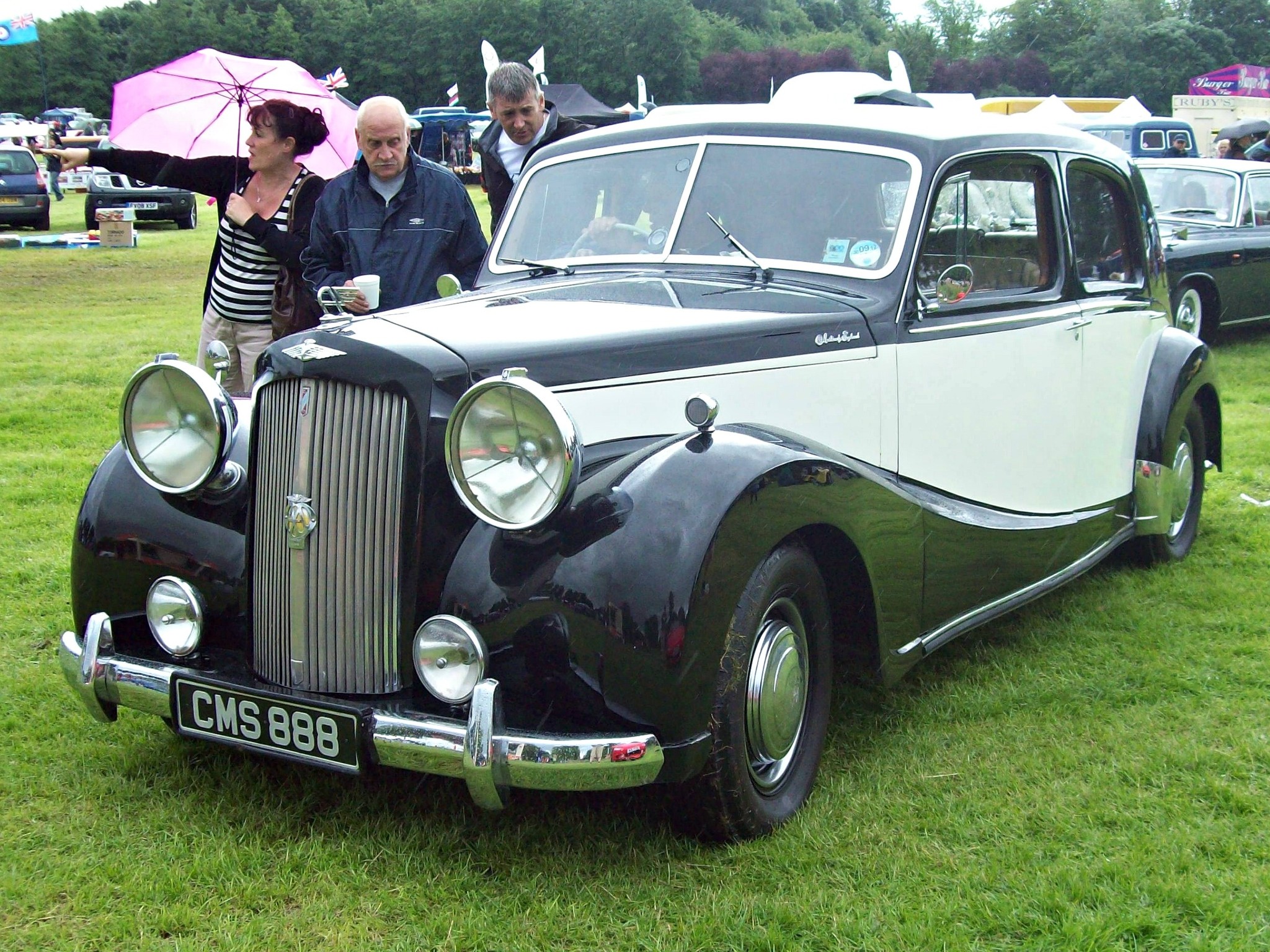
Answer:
[352,274,380,310]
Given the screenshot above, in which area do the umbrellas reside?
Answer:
[107,46,365,260]
[41,106,71,121]
[854,87,934,108]
[1212,116,1270,142]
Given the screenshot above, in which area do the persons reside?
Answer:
[299,96,487,319]
[477,61,598,246]
[567,169,729,258]
[1162,133,1190,158]
[42,120,107,202]
[1212,115,1270,163]
[411,126,483,185]
[39,99,333,399]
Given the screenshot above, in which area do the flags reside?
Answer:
[316,66,350,91]
[0,11,39,47]
[444,83,460,103]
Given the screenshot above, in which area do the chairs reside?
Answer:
[922,254,1041,291]
[0,159,12,171]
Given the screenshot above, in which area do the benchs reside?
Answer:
[876,225,1042,265]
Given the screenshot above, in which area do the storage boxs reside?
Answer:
[99,221,133,247]
[95,207,137,221]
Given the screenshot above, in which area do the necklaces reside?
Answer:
[253,173,290,205]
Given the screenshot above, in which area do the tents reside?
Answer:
[537,84,631,127]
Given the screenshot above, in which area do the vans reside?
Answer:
[0,113,26,125]
[1078,116,1199,158]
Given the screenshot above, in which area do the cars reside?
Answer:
[0,145,51,230]
[1133,158,1270,347]
[84,137,196,231]
[61,111,1224,838]
[55,135,107,189]
